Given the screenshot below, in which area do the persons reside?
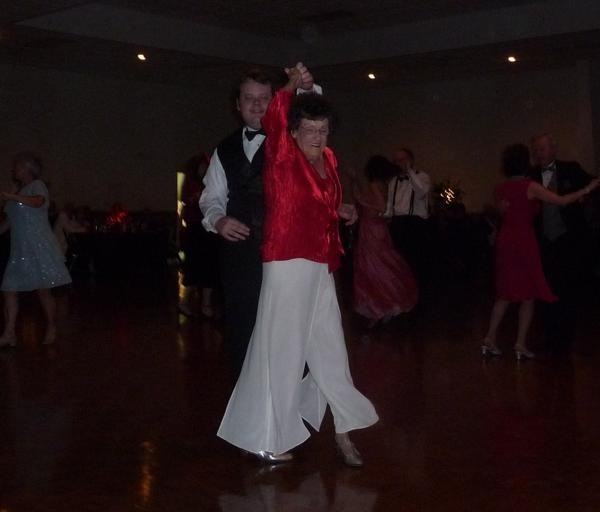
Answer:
[351,156,418,332]
[481,135,600,360]
[0,160,73,347]
[217,68,379,467]
[532,137,600,240]
[199,62,322,365]
[383,149,431,264]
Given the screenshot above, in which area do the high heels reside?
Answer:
[480,344,502,355]
[268,453,293,461]
[335,433,364,467]
[514,349,535,360]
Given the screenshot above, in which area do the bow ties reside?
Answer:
[543,165,555,172]
[397,175,409,182]
[245,128,266,141]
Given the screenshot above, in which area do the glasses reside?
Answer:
[305,128,330,136]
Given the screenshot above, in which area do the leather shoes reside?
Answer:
[0,334,19,347]
[41,328,58,345]
